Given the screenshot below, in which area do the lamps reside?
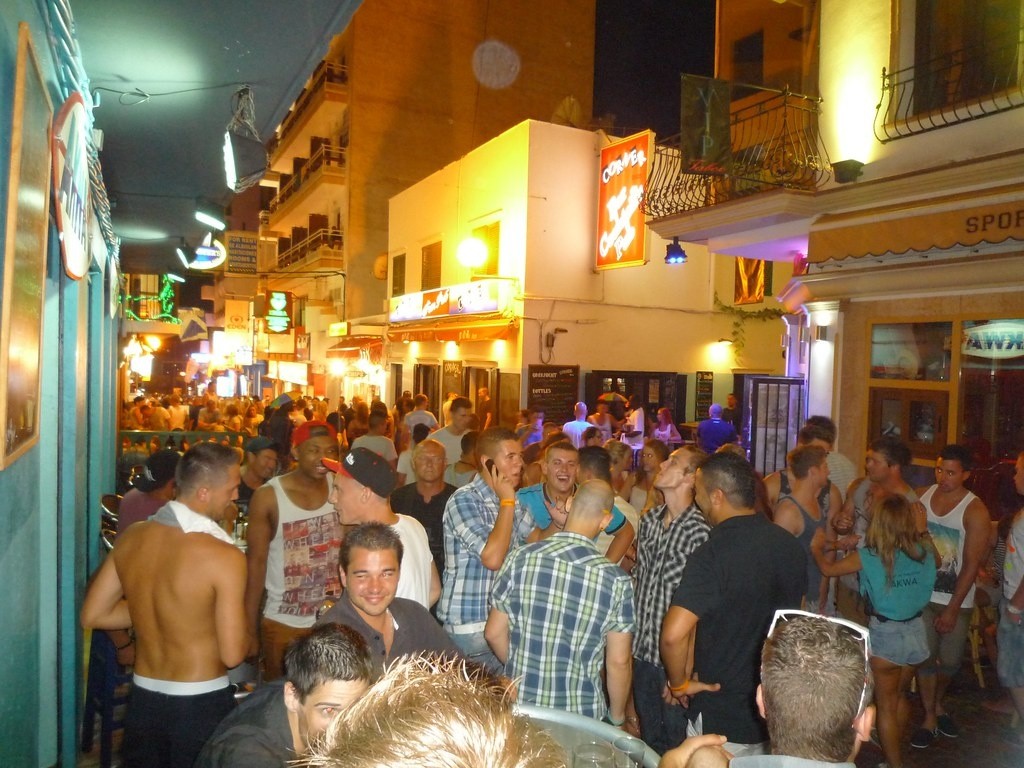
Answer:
[664,234,689,264]
[718,338,733,346]
[175,238,198,269]
[221,131,269,194]
[554,327,569,334]
[194,197,229,231]
[829,159,866,183]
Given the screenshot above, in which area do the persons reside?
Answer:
[118,382,1024,768]
[79,442,247,768]
[285,650,735,768]
[312,520,471,682]
[686,609,877,768]
[659,452,808,757]
[483,479,640,729]
[630,444,713,757]
[315,447,441,618]
[436,425,569,678]
[242,420,357,683]
[193,622,370,768]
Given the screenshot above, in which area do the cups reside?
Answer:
[573,743,614,768]
[613,737,645,768]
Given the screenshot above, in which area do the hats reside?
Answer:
[322,447,398,498]
[246,437,280,453]
[131,449,181,492]
[288,421,339,463]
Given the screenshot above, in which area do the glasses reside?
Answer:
[767,609,871,728]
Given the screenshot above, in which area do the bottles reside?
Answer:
[150,436,160,454]
[532,419,542,432]
[196,437,204,447]
[236,509,244,539]
[223,436,231,447]
[166,436,176,452]
[208,437,217,443]
[236,436,245,448]
[181,436,189,453]
[135,438,146,455]
[243,516,248,539]
[123,437,130,455]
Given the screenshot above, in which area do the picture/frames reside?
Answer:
[0,19,53,471]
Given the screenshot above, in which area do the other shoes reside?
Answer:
[910,728,940,748]
[1001,727,1024,748]
[935,713,959,737]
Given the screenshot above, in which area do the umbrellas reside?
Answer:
[597,391,628,409]
[270,390,303,410]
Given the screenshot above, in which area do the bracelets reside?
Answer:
[501,499,515,506]
[832,543,835,549]
[316,604,330,619]
[1006,603,1024,614]
[667,680,689,692]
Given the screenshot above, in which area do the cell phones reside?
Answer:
[485,460,499,477]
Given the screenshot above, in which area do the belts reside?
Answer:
[443,621,487,635]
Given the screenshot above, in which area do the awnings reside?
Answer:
[326,339,382,359]
